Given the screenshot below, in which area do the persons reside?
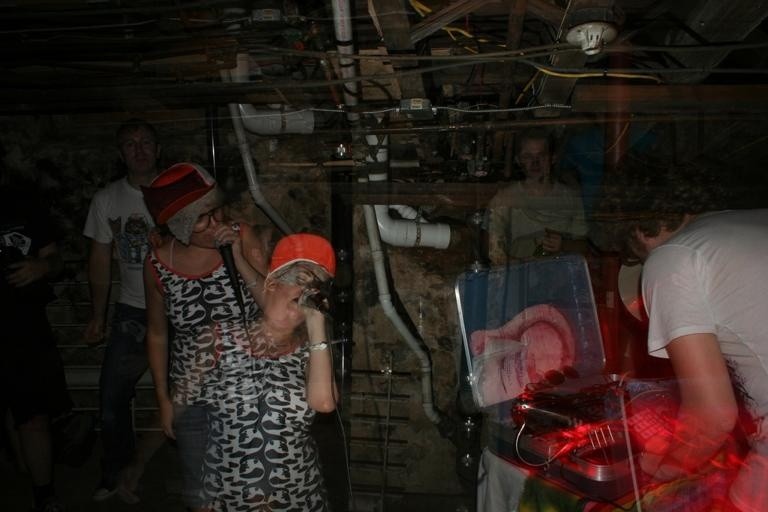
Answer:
[114,231,341,512]
[456,125,767,510]
[0,183,77,512]
[79,117,174,503]
[137,161,272,511]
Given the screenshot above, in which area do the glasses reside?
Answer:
[193,200,233,233]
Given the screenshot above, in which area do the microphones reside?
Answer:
[215,236,245,315]
[302,294,329,317]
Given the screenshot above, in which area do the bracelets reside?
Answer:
[307,341,329,352]
[243,271,261,290]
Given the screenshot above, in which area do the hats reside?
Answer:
[139,163,216,223]
[265,233,336,280]
